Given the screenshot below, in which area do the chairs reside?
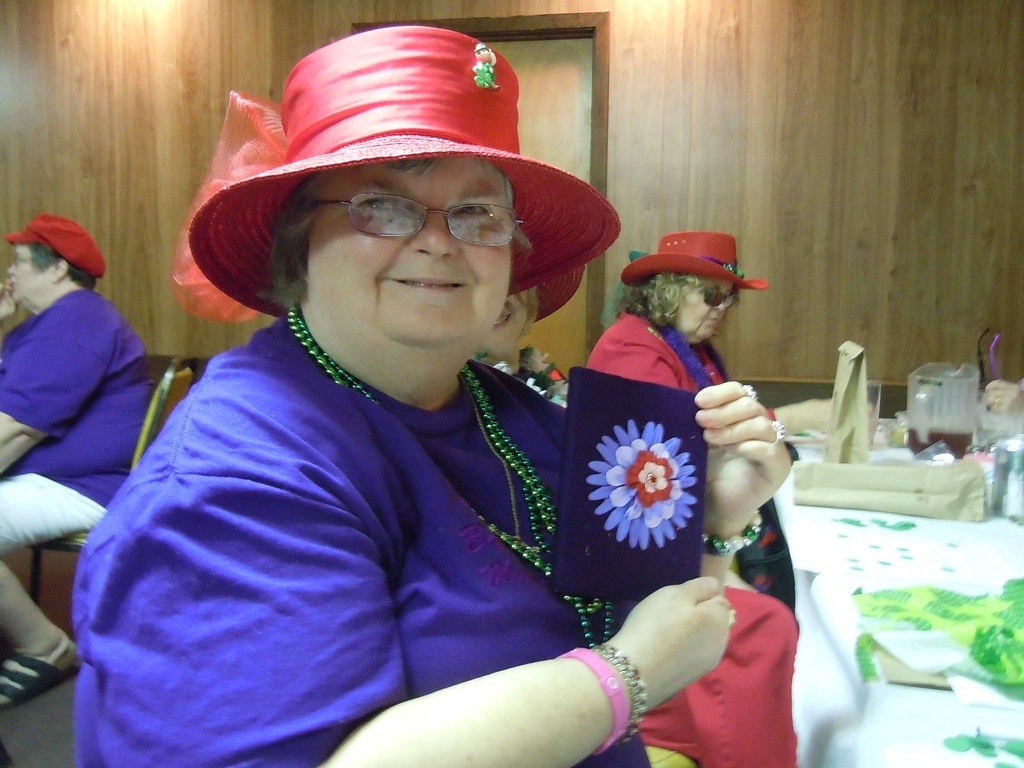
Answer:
[28,356,199,604]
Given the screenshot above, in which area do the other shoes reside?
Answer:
[0,631,78,710]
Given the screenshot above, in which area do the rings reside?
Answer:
[769,420,786,444]
[993,398,1000,408]
[742,385,758,400]
[728,607,736,626]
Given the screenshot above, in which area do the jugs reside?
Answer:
[905,362,981,459]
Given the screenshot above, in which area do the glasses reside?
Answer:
[699,288,734,306]
[301,191,523,246]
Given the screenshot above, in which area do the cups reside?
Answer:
[867,381,881,451]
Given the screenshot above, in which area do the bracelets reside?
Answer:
[593,644,649,746]
[557,646,630,757]
[702,509,764,557]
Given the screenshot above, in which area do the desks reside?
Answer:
[772,416,1024,768]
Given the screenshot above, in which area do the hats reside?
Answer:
[534,265,583,321]
[5,214,105,279]
[621,232,768,290]
[189,26,620,317]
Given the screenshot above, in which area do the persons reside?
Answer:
[585,231,770,395]
[473,263,800,768]
[70,22,792,768]
[982,379,1024,413]
[1,212,156,707]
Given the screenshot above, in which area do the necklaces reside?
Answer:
[472,345,569,409]
[286,307,614,649]
